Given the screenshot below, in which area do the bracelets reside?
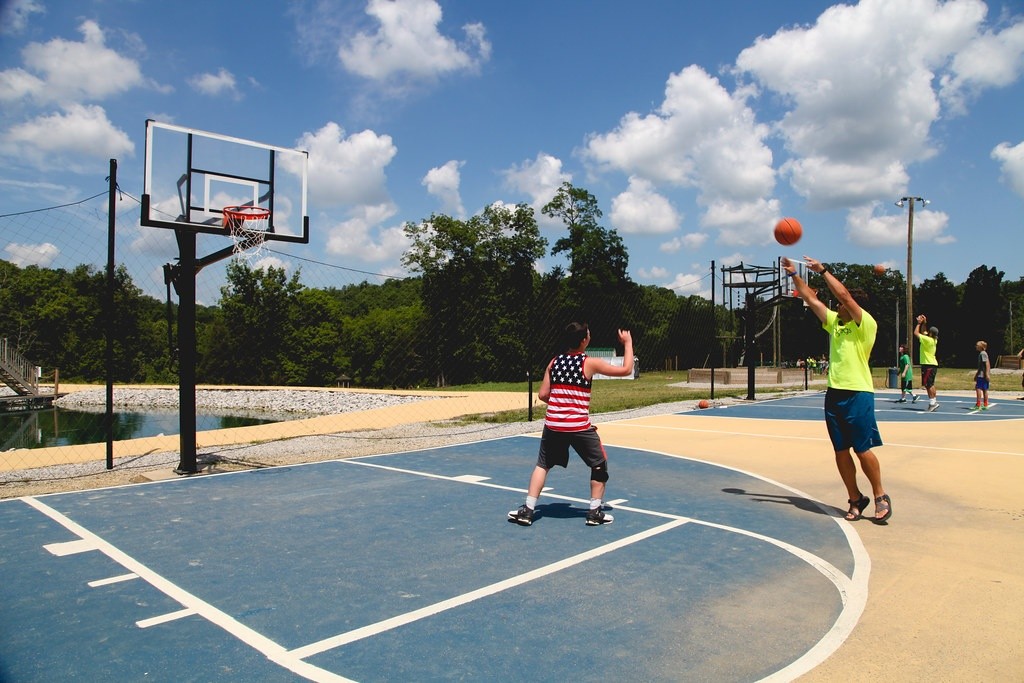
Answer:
[918,323,921,324]
[820,268,827,275]
[787,271,797,277]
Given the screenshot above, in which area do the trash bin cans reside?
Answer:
[889,368,898,388]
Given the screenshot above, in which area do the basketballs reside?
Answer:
[874,264,885,274]
[699,400,708,409]
[774,217,802,246]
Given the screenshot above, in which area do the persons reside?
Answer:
[784,355,829,375]
[780,255,892,523]
[914,315,940,412]
[508,322,634,525]
[895,345,920,403]
[969,341,990,410]
[1018,349,1024,400]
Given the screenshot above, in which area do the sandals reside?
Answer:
[845,493,870,521]
[875,494,892,523]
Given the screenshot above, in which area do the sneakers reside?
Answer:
[927,402,939,411]
[912,395,920,403]
[981,405,989,410]
[586,505,614,524]
[508,504,533,525]
[895,399,907,404]
[969,405,982,411]
